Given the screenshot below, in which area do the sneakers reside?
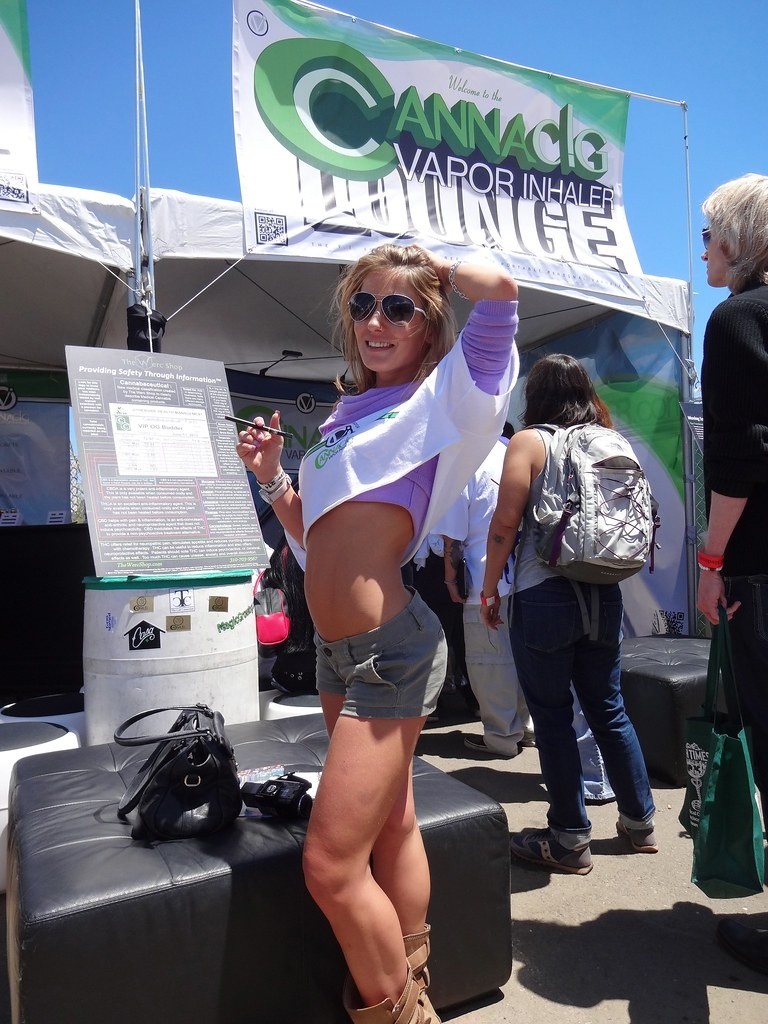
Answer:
[463,735,518,755]
[523,741,536,746]
[616,821,659,852]
[510,828,594,874]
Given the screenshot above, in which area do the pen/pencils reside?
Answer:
[223,415,293,440]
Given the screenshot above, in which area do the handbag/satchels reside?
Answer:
[271,645,319,695]
[258,640,291,658]
[113,702,242,841]
[254,571,291,645]
[679,604,765,900]
[436,670,480,723]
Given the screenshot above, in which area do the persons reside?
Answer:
[235,243,521,1024]
[695,172,768,980]
[265,354,660,873]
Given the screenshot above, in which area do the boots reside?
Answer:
[403,923,442,1024]
[344,958,440,1024]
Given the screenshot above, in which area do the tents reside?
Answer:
[0,179,702,705]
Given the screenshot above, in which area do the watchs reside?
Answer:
[259,475,292,506]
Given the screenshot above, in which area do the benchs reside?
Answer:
[3,703,514,1024]
[615,631,730,789]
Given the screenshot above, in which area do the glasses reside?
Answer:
[347,291,434,326]
[701,228,710,250]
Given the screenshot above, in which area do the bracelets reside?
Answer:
[695,547,724,574]
[257,468,286,493]
[448,261,473,301]
[480,590,501,607]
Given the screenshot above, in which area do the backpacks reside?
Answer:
[531,423,662,584]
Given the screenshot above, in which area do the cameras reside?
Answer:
[241,776,312,823]
